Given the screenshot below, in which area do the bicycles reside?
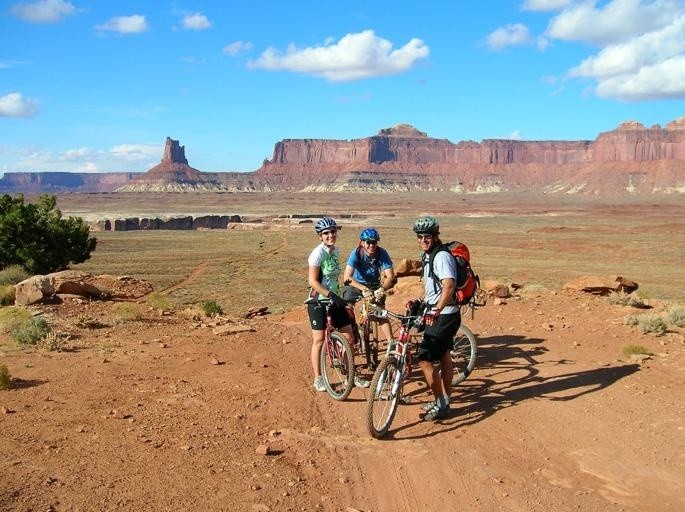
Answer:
[356,294,476,386]
[312,293,354,401]
[367,300,439,440]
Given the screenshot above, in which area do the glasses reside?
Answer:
[323,230,337,234]
[367,241,377,244]
[416,234,433,239]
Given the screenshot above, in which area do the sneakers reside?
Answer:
[345,376,370,388]
[421,402,451,421]
[313,375,328,392]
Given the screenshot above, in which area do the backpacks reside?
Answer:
[421,241,480,305]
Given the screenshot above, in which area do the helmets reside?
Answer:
[413,216,442,234]
[315,217,342,233]
[360,228,381,241]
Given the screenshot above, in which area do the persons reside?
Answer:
[412,214,463,423]
[341,227,395,352]
[306,217,372,392]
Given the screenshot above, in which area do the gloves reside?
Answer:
[328,291,344,309]
